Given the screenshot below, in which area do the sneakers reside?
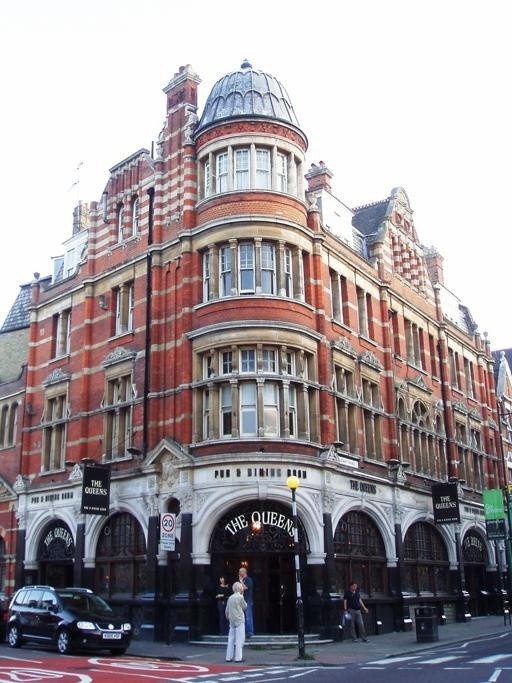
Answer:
[352,637,370,643]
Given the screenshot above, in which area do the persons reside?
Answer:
[342,580,371,643]
[237,567,255,639]
[215,571,232,637]
[223,579,248,663]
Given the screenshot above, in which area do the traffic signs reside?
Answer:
[161,512,176,550]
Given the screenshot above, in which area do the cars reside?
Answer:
[6,585,133,656]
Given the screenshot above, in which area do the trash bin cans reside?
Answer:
[414,606,439,643]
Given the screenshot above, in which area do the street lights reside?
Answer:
[287,475,305,659]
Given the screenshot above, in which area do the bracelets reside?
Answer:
[344,608,347,611]
